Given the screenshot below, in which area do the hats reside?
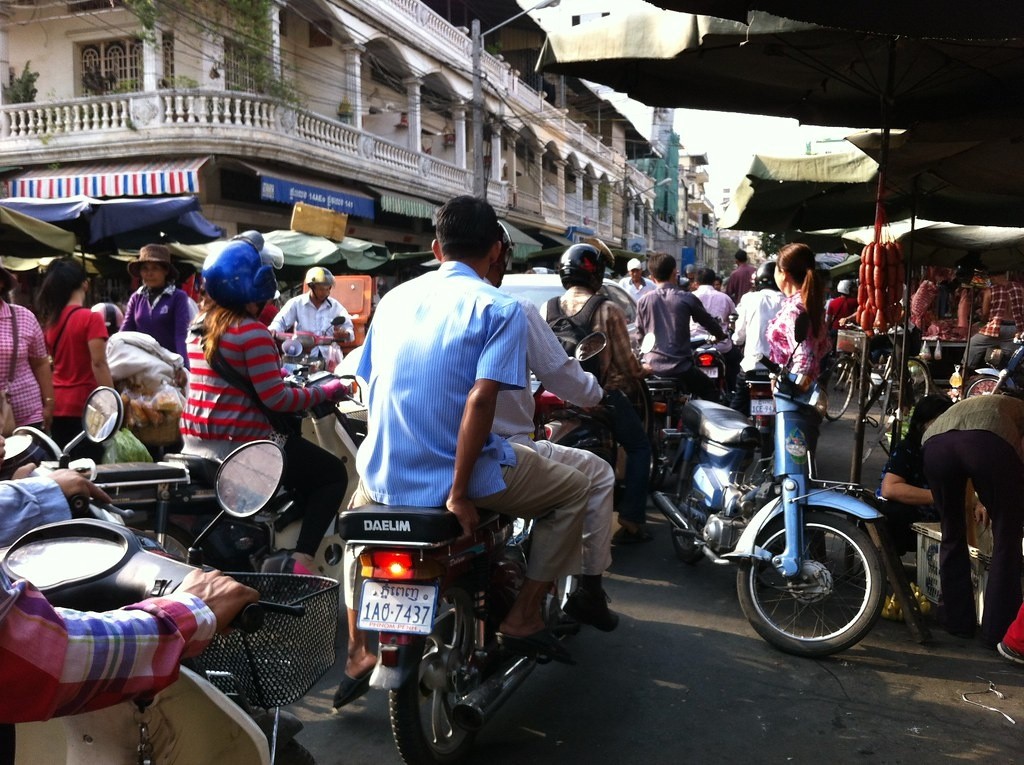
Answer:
[0,257,17,291]
[127,244,179,281]
[627,258,641,271]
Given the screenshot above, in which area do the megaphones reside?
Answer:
[281,339,302,357]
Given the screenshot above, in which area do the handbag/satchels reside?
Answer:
[0,390,16,437]
[258,402,302,439]
[102,427,153,464]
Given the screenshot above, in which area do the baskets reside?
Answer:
[177,572,340,708]
[836,328,865,352]
[282,354,326,372]
[344,407,368,448]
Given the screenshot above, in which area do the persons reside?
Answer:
[0,265,57,435]
[0,435,262,725]
[266,266,357,372]
[824,264,1024,667]
[174,229,358,578]
[39,256,114,465]
[257,298,279,327]
[118,245,190,369]
[333,190,621,706]
[538,243,831,559]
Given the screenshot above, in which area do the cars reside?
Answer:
[491,272,647,420]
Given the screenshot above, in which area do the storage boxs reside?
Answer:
[914,521,994,627]
[290,202,347,243]
[303,275,372,347]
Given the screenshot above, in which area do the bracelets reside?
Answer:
[45,397,54,401]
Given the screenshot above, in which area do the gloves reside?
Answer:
[317,378,348,403]
[279,366,291,380]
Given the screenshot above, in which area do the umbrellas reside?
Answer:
[204,228,390,302]
[535,0,1024,449]
[0,194,226,280]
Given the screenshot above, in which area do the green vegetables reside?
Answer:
[99,430,154,464]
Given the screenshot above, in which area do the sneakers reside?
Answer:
[997,642,1024,664]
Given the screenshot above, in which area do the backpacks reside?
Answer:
[545,293,611,386]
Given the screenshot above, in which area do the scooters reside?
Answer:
[963,332,1024,403]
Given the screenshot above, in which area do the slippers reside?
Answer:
[335,666,379,708]
[496,623,576,666]
[618,526,654,544]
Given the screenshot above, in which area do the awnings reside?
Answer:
[538,231,576,247]
[496,217,543,261]
[7,155,214,200]
[233,158,375,219]
[368,184,438,219]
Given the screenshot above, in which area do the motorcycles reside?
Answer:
[0,422,341,765]
[339,332,624,765]
[64,355,365,582]
[270,315,361,376]
[521,341,892,659]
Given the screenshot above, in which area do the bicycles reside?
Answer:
[822,320,930,423]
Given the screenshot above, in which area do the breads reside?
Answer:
[119,384,186,426]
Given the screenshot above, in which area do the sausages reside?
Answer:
[856,242,905,337]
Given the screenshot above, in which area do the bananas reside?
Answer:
[881,582,931,621]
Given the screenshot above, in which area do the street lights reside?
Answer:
[621,177,672,250]
[470,0,563,196]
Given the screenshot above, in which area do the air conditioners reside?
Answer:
[624,237,647,255]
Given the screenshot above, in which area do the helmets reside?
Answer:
[559,242,606,291]
[490,221,513,286]
[837,278,855,296]
[755,262,778,290]
[202,230,284,313]
[686,264,696,274]
[304,265,336,288]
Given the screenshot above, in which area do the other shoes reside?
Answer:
[288,560,334,589]
[932,604,972,637]
[978,631,999,650]
[562,585,618,631]
